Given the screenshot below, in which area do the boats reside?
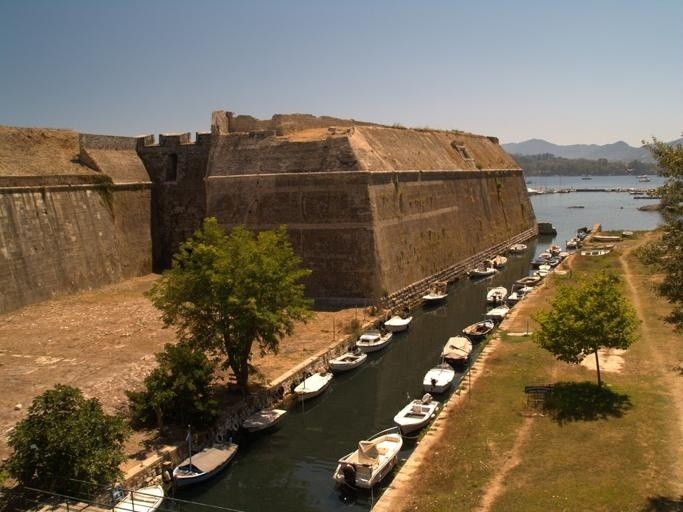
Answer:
[171,424,240,488]
[592,234,621,242]
[422,281,449,303]
[332,426,404,489]
[636,173,651,183]
[422,362,455,395]
[468,226,591,308]
[240,406,289,435]
[462,320,495,340]
[580,249,612,256]
[108,484,165,512]
[294,366,334,401]
[328,347,368,373]
[582,177,592,180]
[485,305,510,321]
[355,328,393,353]
[393,392,440,436]
[439,333,473,363]
[383,315,413,333]
